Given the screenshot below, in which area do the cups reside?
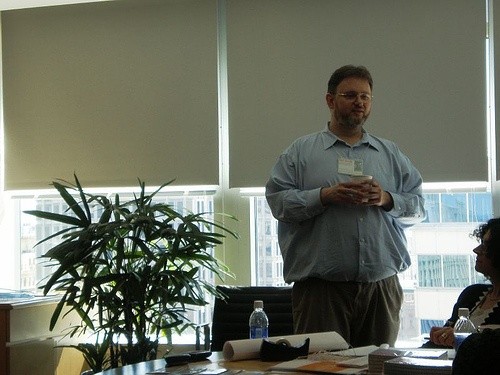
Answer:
[351,174,373,203]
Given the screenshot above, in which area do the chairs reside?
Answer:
[197,284,298,353]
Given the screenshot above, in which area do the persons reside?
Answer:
[265,65,427,348]
[421,216,500,350]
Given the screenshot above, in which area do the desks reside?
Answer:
[89,342,455,375]
[0,290,97,375]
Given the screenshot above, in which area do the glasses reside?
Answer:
[330,91,374,100]
[481,240,489,251]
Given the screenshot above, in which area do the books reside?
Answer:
[369,349,448,372]
[383,357,453,375]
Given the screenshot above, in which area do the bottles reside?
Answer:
[453,307,478,358]
[249,300,269,340]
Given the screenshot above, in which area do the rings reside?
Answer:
[441,332,447,338]
